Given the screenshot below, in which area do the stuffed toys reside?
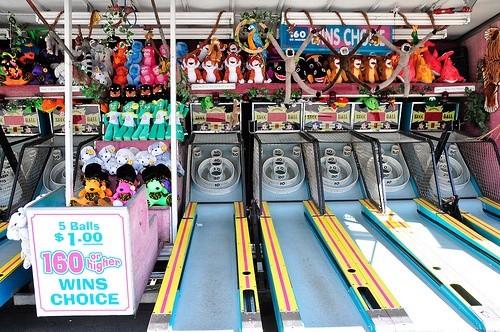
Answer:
[0,20,435,126]
[475,27,500,112]
[419,39,454,77]
[435,58,464,83]
[108,83,181,105]
[70,162,180,207]
[80,141,186,175]
[103,99,189,141]
[6,207,32,269]
